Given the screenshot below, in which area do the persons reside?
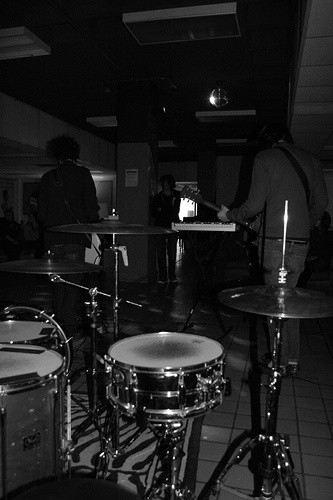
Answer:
[217,124,328,376]
[0,208,39,263]
[36,134,101,349]
[1,190,10,216]
[152,173,180,284]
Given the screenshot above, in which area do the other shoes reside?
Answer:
[158,279,178,285]
[242,362,298,383]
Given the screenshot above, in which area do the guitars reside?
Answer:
[181,186,262,245]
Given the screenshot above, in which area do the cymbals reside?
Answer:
[217,284,333,319]
[45,220,179,234]
[0,258,103,274]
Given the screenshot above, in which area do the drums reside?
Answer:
[0,343,67,499]
[0,305,70,376]
[105,329,226,423]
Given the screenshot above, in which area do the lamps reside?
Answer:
[0,2,259,128]
